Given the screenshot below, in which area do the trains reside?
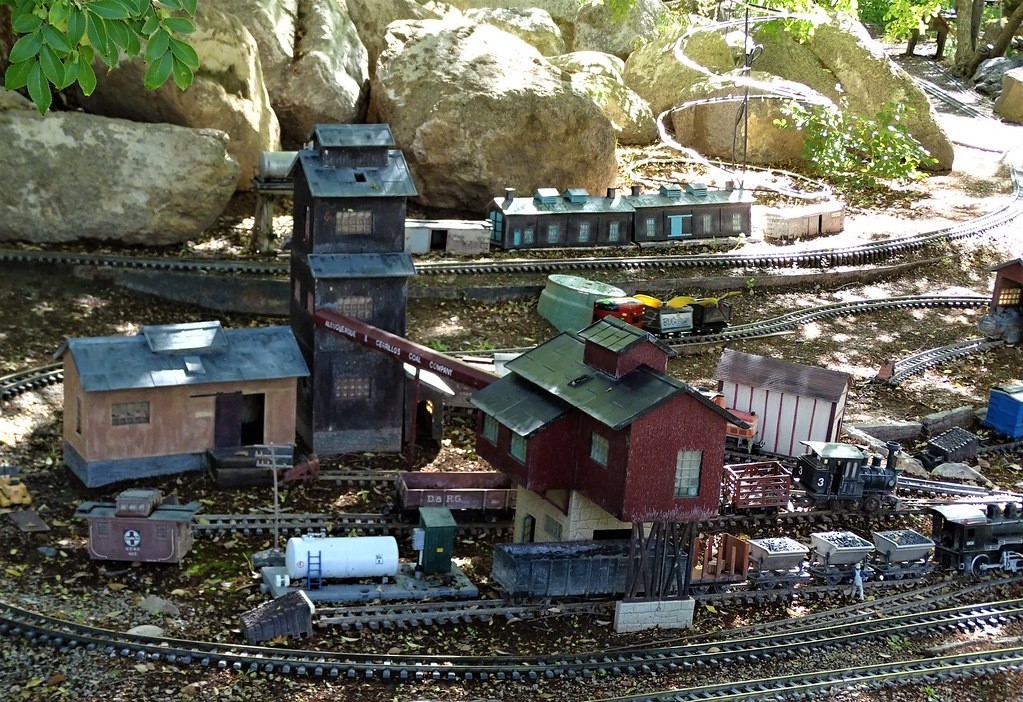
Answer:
[488,501,1023,606]
[723,441,901,518]
[589,293,733,339]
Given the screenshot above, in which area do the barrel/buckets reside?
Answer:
[285,536,399,578]
[257,149,299,177]
